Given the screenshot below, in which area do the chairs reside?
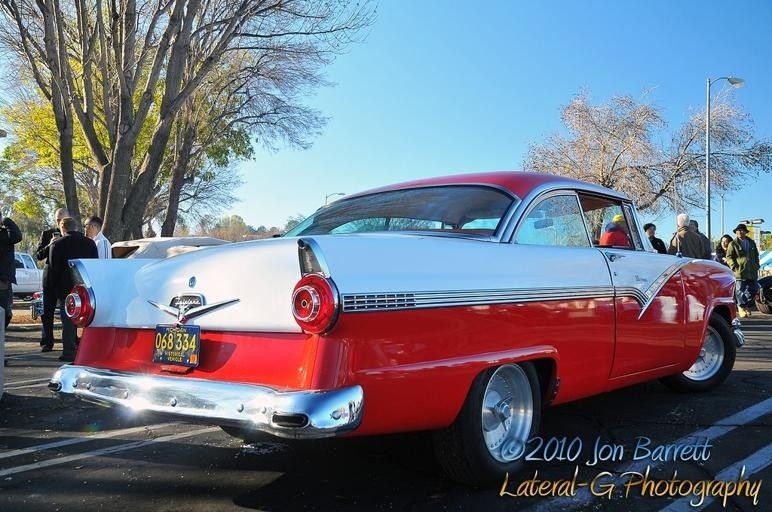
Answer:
[41,344,52,352]
[56,357,73,363]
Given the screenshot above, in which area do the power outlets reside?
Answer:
[10,251,44,301]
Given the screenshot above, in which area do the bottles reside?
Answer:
[644,223,656,232]
[613,215,625,222]
[733,224,749,233]
[677,214,690,227]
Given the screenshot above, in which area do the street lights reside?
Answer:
[704,76,746,248]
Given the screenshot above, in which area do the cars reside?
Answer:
[33,169,746,483]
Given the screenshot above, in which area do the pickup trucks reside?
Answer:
[10,251,44,301]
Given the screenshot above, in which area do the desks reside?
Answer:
[704,76,746,248]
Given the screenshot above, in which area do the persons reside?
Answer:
[689,220,711,259]
[715,235,733,265]
[668,214,704,258]
[726,224,760,317]
[36,208,112,361]
[643,223,667,254]
[0,212,22,329]
[599,214,629,246]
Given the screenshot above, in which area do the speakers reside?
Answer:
[738,306,751,318]
